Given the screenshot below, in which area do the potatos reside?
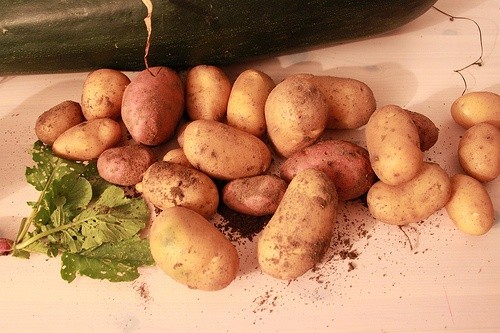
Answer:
[36,65,500,289]
